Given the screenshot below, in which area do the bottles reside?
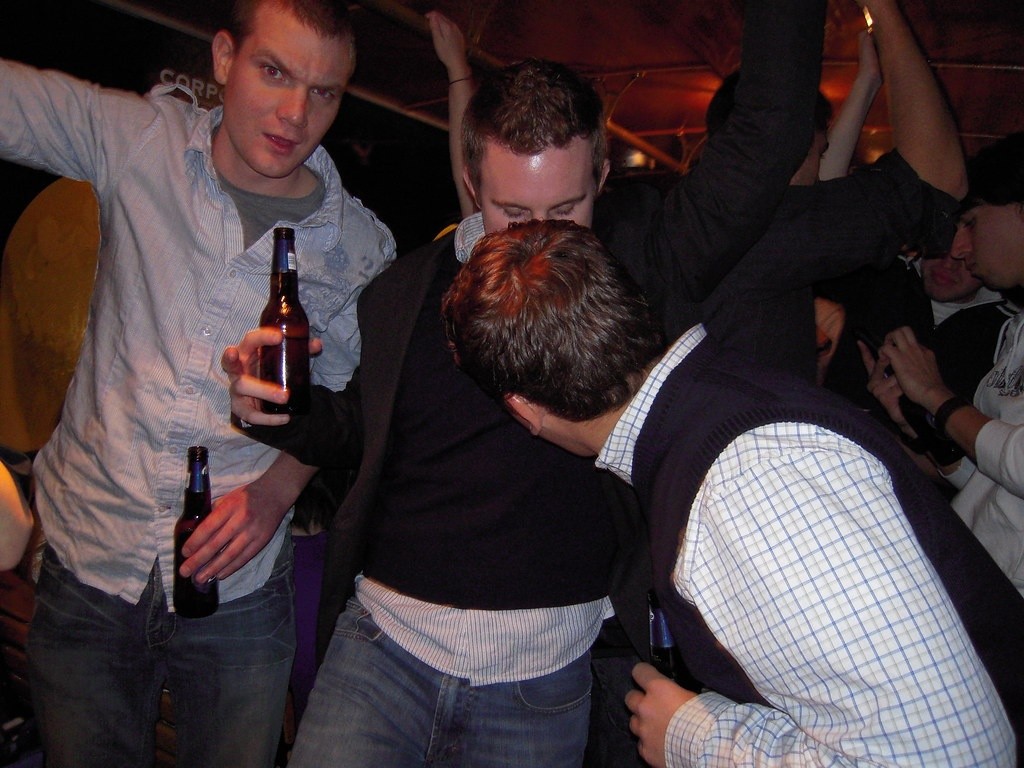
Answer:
[648,588,681,686]
[173,446,220,619]
[259,227,308,425]
[851,322,969,468]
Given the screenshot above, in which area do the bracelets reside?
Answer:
[932,396,972,431]
[903,434,926,459]
[448,78,472,86]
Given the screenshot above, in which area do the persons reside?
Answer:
[1,1,1022,768]
